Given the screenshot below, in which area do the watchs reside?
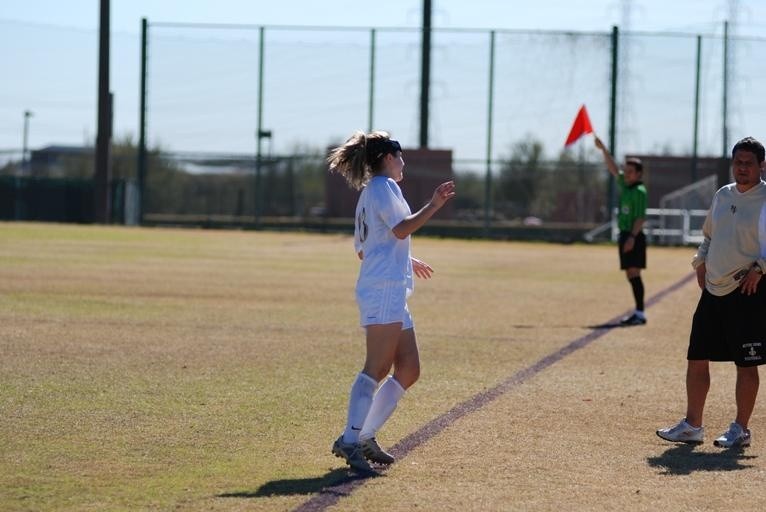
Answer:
[751,262,765,276]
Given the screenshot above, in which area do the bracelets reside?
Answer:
[629,232,636,238]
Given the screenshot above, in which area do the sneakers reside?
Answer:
[654,416,706,445]
[712,420,752,449]
[360,435,395,466]
[620,313,648,326]
[331,433,375,476]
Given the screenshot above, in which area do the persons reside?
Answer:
[592,138,651,325]
[327,130,456,482]
[655,133,766,448]
